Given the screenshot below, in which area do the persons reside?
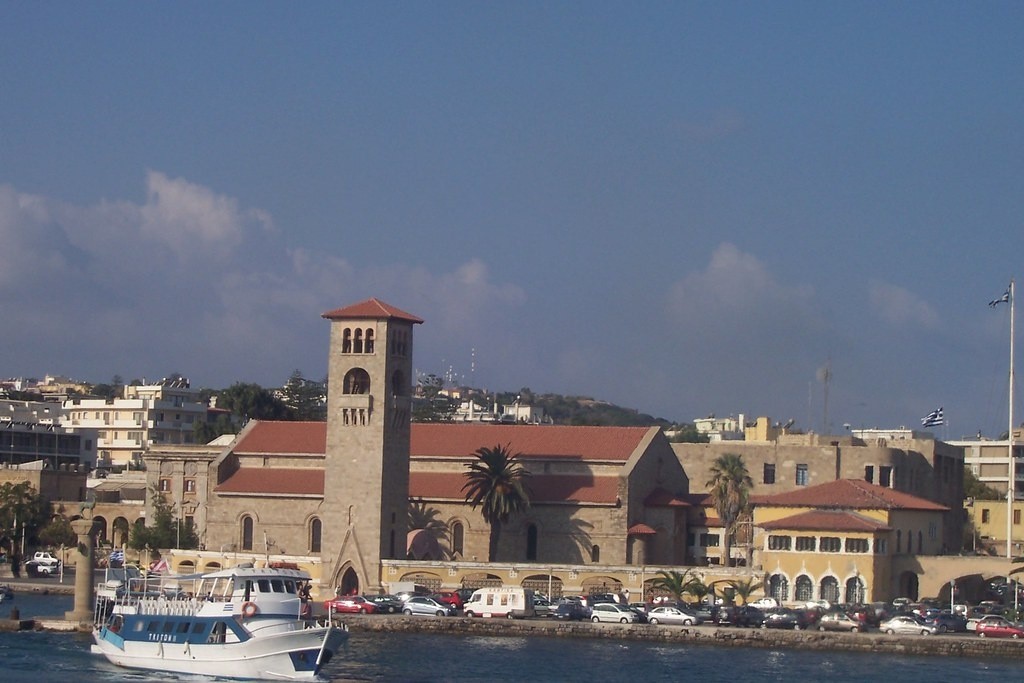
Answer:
[335,585,341,597]
[297,585,313,603]
[207,592,213,602]
[625,590,630,600]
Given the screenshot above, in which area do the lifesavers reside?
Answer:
[241,601,257,617]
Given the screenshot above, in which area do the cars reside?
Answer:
[976,619,1024,639]
[762,609,808,631]
[645,606,705,626]
[325,588,478,616]
[590,604,638,625]
[554,603,584,623]
[34,550,61,566]
[25,560,56,573]
[818,612,863,633]
[532,584,1024,632]
[878,616,941,635]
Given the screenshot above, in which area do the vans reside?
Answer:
[462,586,536,620]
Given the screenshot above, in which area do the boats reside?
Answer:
[91,545,350,683]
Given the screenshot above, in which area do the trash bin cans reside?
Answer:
[26,564,38,578]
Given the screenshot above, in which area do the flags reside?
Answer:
[921,407,944,428]
[989,283,1011,310]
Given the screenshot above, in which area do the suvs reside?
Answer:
[715,605,749,629]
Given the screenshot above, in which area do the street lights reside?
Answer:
[950,578,956,613]
[855,570,860,604]
[1015,576,1020,610]
